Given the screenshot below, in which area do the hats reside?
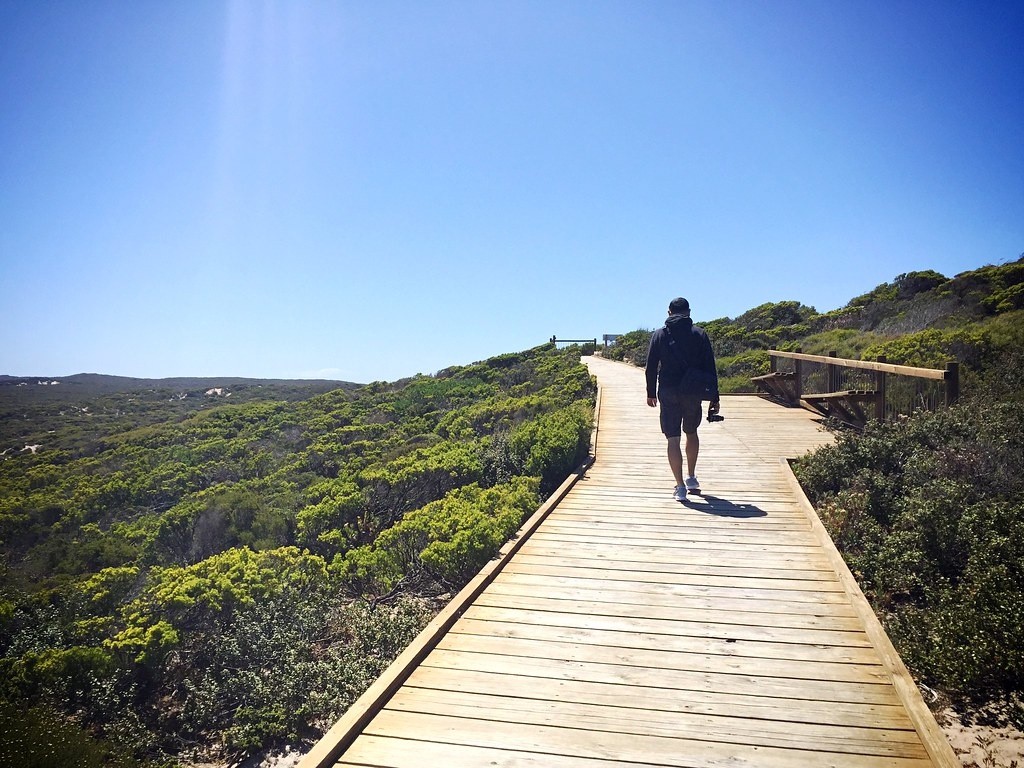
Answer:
[669,297,689,315]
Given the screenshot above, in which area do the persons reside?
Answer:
[645,298,720,500]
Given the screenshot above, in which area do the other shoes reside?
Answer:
[673,485,686,500]
[686,478,700,489]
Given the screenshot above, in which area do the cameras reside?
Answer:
[707,410,724,422]
